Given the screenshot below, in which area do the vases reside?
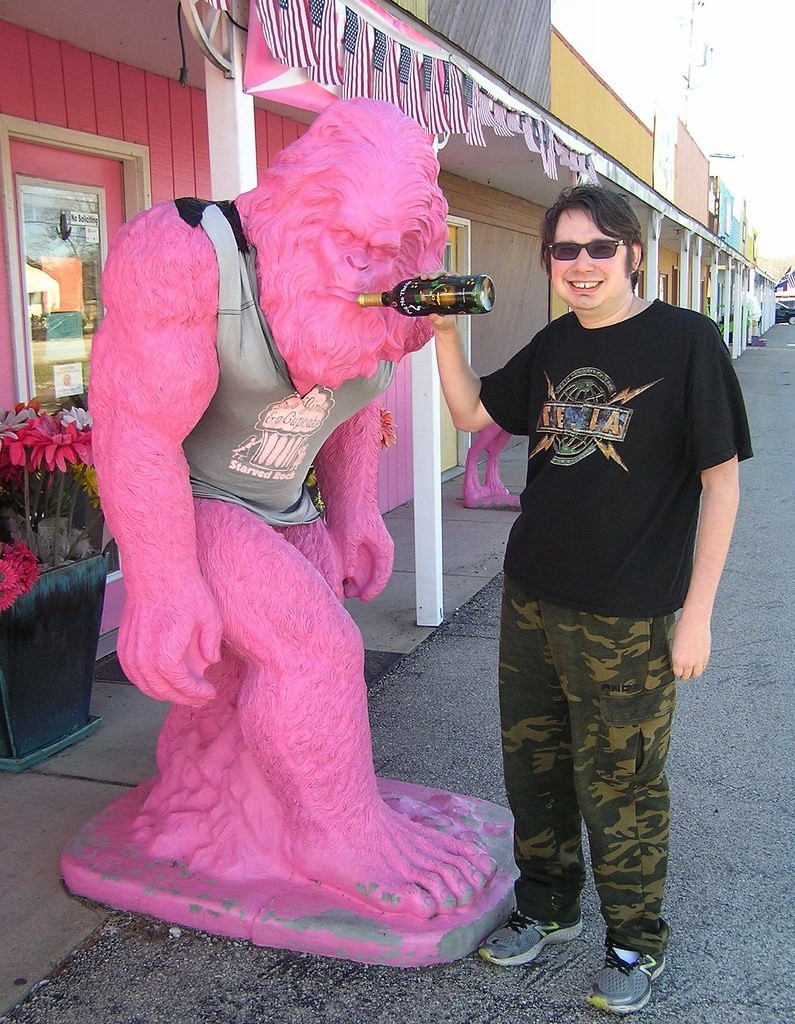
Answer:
[0,549,112,775]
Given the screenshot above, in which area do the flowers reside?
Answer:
[0,395,103,612]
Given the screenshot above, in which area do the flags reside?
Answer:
[252,0,599,185]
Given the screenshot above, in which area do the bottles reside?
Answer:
[360,275,496,316]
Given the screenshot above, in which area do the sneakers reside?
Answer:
[478,909,583,966]
[585,935,667,1014]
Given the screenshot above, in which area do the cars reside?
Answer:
[760,301,795,326]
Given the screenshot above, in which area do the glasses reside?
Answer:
[545,237,625,260]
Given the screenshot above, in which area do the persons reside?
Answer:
[434,182,752,1015]
